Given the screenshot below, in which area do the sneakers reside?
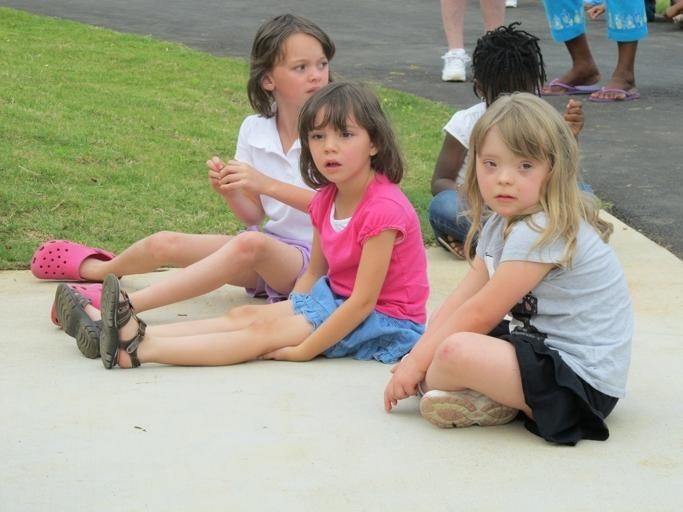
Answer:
[420,388,520,428]
[505,0,517,8]
[442,48,466,82]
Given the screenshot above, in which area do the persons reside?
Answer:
[384,94,634,448]
[32,15,336,327]
[432,22,583,259]
[542,0,645,104]
[53,78,430,369]
[438,0,513,82]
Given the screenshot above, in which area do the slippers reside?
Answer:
[589,84,640,102]
[535,78,602,96]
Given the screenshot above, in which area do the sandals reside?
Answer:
[31,240,124,283]
[100,274,145,368]
[51,283,103,326]
[55,285,103,358]
[437,236,465,259]
